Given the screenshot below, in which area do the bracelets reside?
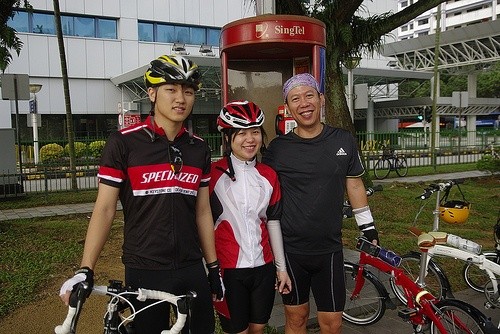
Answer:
[207,259,220,268]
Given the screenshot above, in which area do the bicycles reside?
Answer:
[54,280,197,334]
[374,144,408,180]
[342,179,500,334]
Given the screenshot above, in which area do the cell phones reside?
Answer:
[355,238,381,258]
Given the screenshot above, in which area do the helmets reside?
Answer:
[144,54,202,91]
[439,200,471,225]
[217,100,265,132]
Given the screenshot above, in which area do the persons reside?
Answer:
[209,101,292,334]
[59,54,225,334]
[262,73,380,334]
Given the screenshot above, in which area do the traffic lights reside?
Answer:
[416,108,424,122]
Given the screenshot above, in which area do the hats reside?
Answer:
[283,73,320,102]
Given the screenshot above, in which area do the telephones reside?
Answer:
[275,105,298,136]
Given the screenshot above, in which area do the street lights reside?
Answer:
[30,84,43,167]
[340,57,362,125]
